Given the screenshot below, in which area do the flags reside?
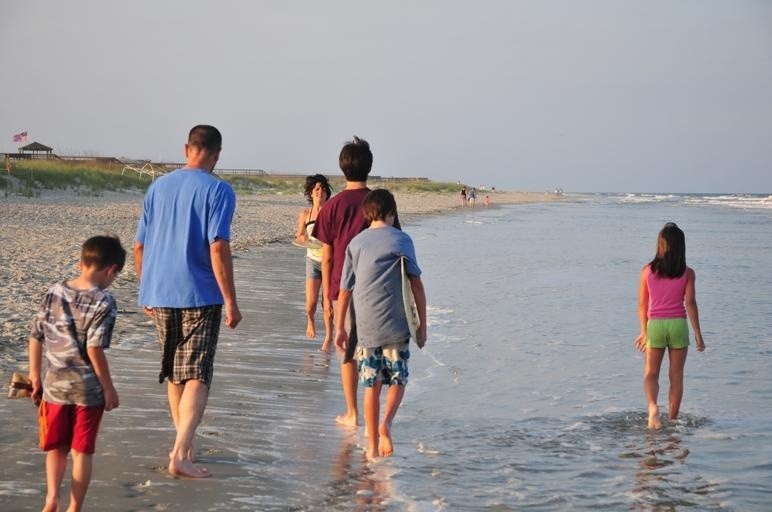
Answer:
[13,132,28,142]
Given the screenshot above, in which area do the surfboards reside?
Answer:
[401,257,420,342]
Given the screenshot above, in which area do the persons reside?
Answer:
[27,234,127,512]
[133,125,243,478]
[310,136,401,438]
[459,185,495,209]
[634,222,705,429]
[296,174,335,353]
[334,189,427,458]
